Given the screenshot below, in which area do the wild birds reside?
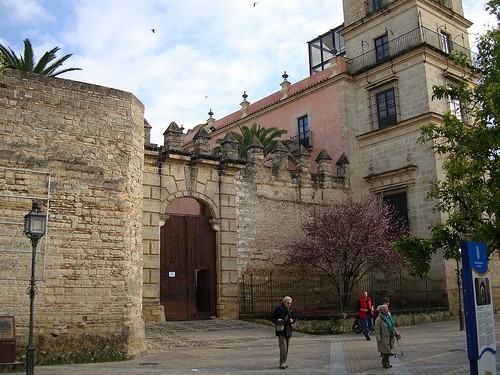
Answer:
[151,29,155,33]
[205,96,208,98]
[254,2,255,7]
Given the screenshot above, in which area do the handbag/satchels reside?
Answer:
[275,319,285,332]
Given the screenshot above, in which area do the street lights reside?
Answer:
[23,199,47,374]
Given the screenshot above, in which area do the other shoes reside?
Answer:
[389,365,392,367]
[383,365,389,368]
[286,365,289,367]
[280,367,285,369]
[366,336,370,340]
[372,327,375,330]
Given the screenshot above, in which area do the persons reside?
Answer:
[375,297,401,369]
[270,297,293,369]
[356,291,375,340]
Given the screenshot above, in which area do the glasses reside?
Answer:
[287,303,291,304]
